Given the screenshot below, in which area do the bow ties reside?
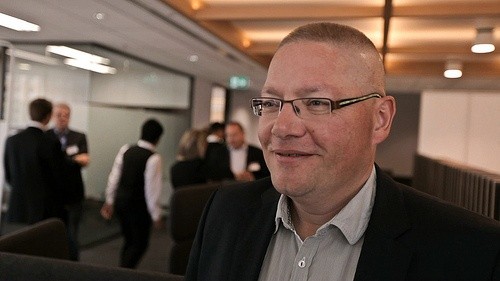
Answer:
[55,128,69,137]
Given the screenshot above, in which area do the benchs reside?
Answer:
[0,217,185,281]
[167,185,213,276]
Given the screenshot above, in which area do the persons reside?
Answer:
[181,22,500,281]
[2,99,90,260]
[169,121,271,273]
[101,118,163,269]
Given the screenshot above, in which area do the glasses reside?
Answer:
[250,94,383,119]
